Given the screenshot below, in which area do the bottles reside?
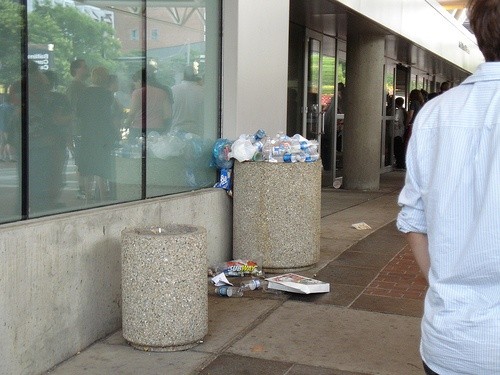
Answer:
[247,128,321,163]
[240,278,268,291]
[208,286,243,298]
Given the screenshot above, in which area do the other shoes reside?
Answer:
[393,165,405,171]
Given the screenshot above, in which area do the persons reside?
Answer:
[0,59,206,215]
[306,81,450,171]
[395,0,500,375]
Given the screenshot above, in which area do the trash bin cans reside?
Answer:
[119,223,211,353]
[112,132,216,201]
[232,129,323,274]
